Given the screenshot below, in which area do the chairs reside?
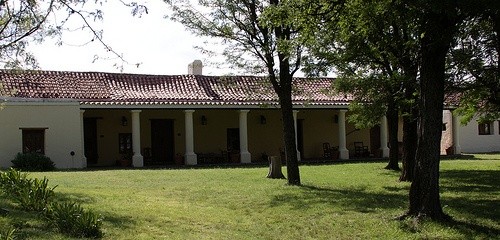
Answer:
[279,148,286,164]
[324,143,333,161]
[354,142,369,160]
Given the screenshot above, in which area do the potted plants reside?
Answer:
[118,158,129,167]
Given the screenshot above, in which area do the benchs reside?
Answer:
[144,147,172,168]
